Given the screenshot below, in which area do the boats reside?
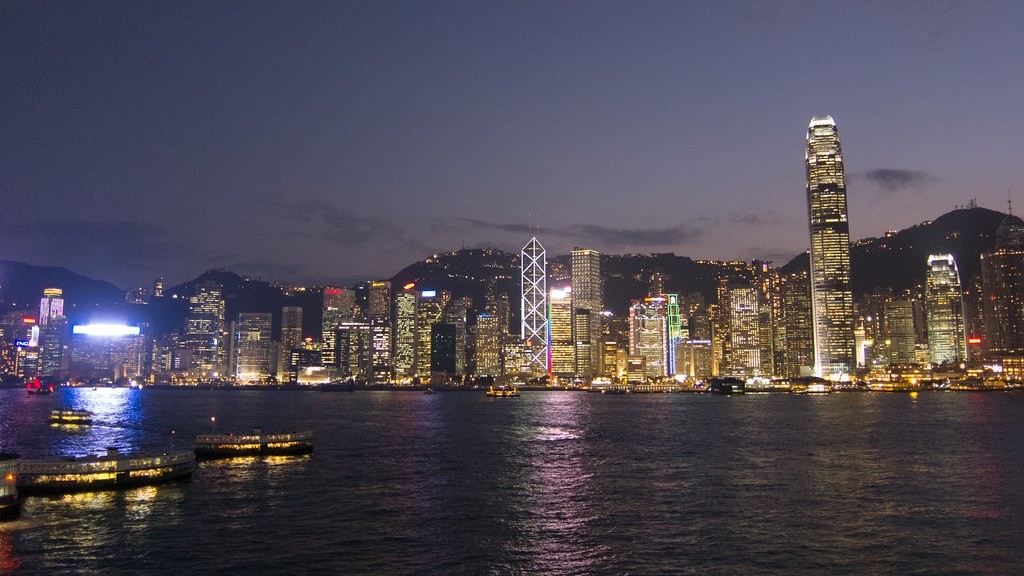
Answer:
[194,431,314,456]
[15,452,195,492]
[486,385,520,397]
[49,409,93,425]
[27,388,50,395]
